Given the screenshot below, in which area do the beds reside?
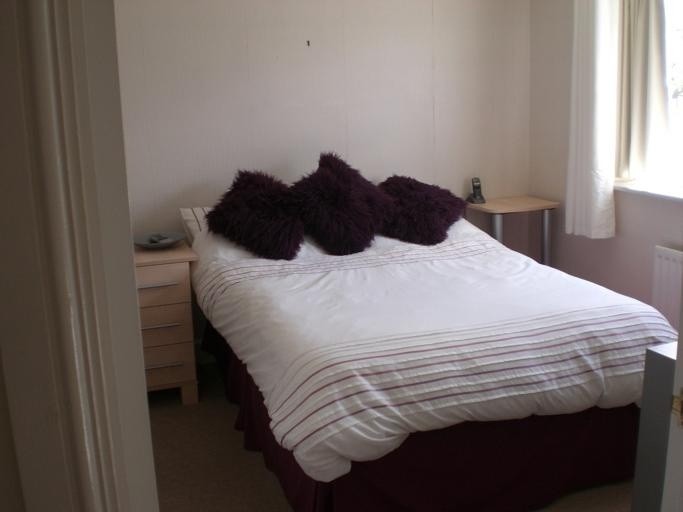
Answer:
[180,187,682,511]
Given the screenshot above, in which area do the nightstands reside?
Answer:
[104,237,199,409]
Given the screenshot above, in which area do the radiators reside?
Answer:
[651,244,683,334]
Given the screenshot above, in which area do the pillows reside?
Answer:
[290,151,397,255]
[205,170,319,261]
[373,174,466,246]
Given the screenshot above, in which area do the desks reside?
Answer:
[467,193,559,267]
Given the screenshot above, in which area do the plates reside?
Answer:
[133,231,188,251]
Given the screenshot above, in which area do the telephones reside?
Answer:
[466,178,485,204]
